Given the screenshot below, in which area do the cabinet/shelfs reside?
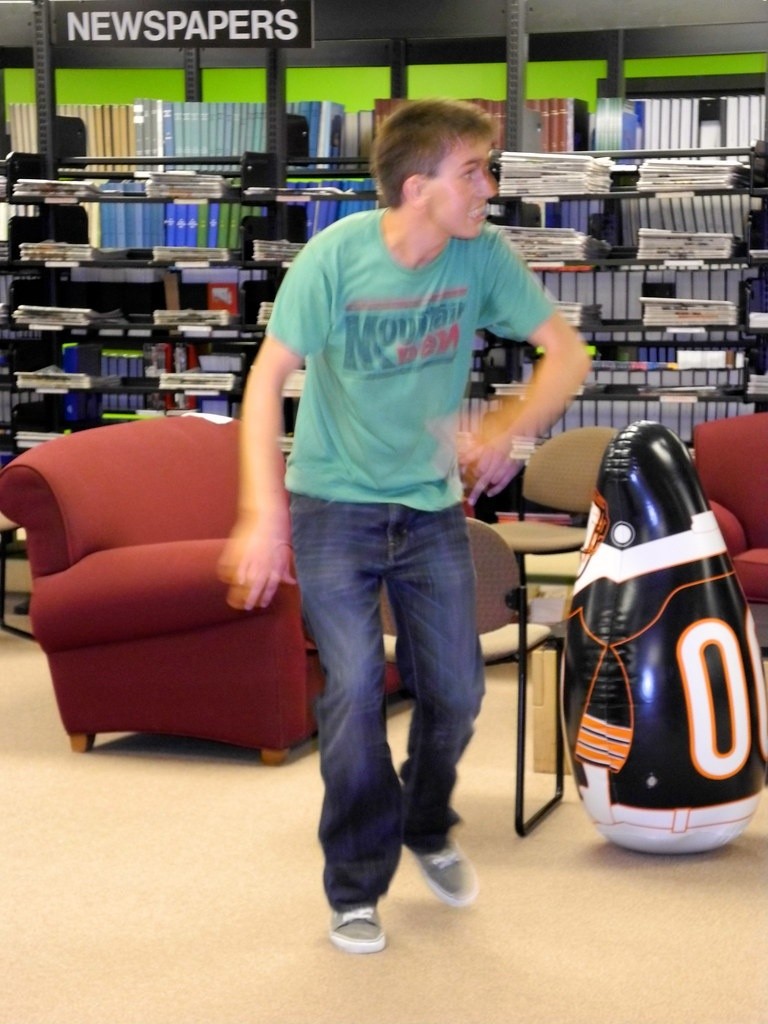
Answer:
[0,147,767,427]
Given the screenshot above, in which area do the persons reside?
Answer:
[217,101,594,950]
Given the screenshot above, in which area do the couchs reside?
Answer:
[0,413,402,761]
[687,412,768,600]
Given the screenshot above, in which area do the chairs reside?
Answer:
[485,427,618,562]
[466,516,564,837]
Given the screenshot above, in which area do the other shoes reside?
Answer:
[328,902,386,955]
[415,829,480,904]
